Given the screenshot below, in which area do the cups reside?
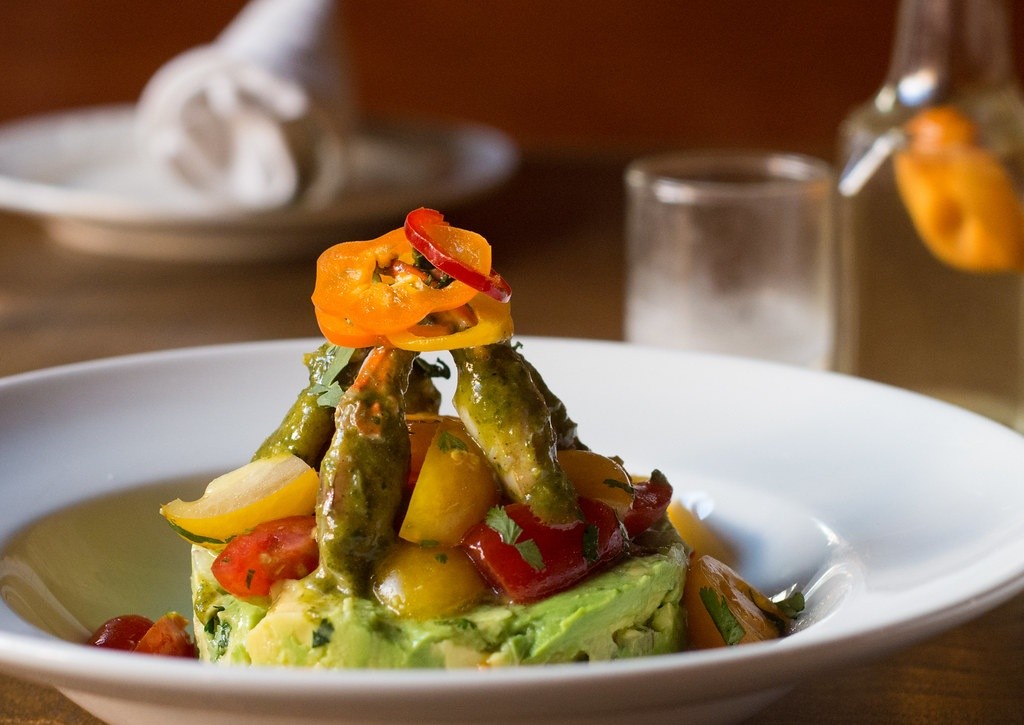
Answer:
[621,150,836,373]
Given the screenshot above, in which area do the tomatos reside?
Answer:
[90,415,782,664]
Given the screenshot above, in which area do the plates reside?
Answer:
[0,336,1022,724]
[2,101,518,261]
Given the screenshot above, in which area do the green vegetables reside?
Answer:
[307,342,750,650]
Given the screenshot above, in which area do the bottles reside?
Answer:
[836,1,1024,436]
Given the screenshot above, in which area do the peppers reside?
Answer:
[310,208,515,353]
